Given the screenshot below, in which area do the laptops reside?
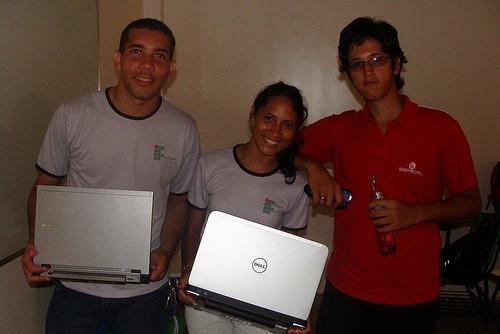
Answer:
[183,211,329,334]
[32,184,153,284]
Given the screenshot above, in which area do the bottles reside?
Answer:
[370,175,396,252]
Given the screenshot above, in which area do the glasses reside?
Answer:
[345,55,394,71]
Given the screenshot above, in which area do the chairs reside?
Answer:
[439,162,500,327]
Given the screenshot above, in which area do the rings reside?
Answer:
[320,196,326,199]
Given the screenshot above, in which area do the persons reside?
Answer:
[176,81,313,334]
[21,17,199,334]
[283,15,479,334]
